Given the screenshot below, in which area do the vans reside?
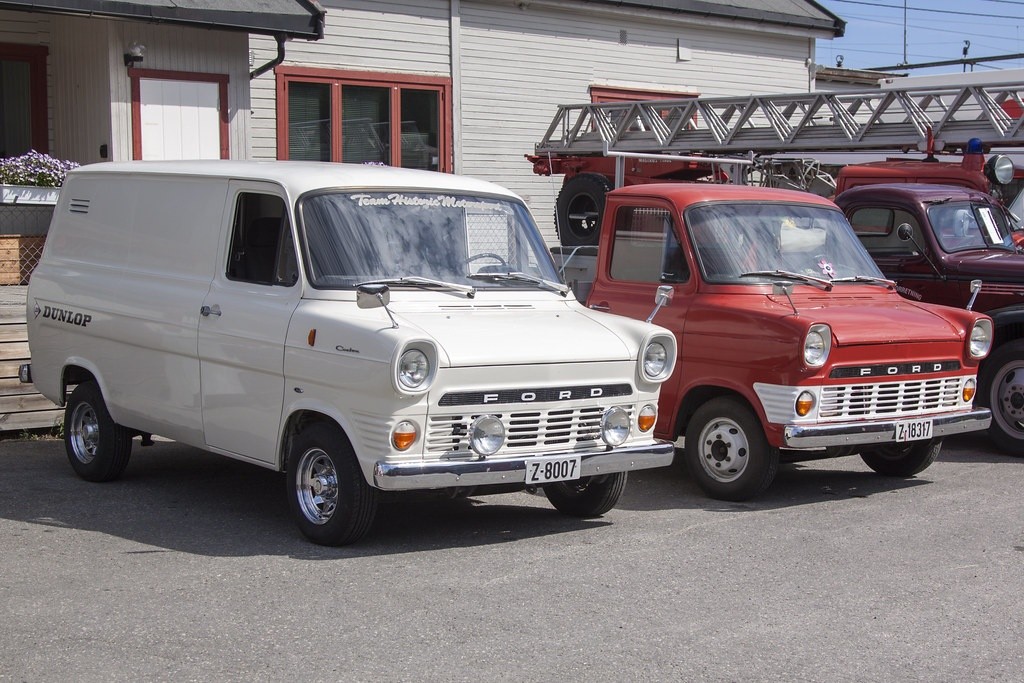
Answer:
[19,160,679,545]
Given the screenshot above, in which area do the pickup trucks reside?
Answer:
[577,182,993,501]
[780,180,1024,457]
[835,139,1023,238]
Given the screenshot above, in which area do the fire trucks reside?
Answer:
[288,121,438,171]
[524,67,1024,304]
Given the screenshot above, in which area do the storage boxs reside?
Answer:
[0,233,47,285]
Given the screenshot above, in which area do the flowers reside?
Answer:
[0,149,82,188]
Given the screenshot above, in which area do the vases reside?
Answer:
[0,184,61,205]
